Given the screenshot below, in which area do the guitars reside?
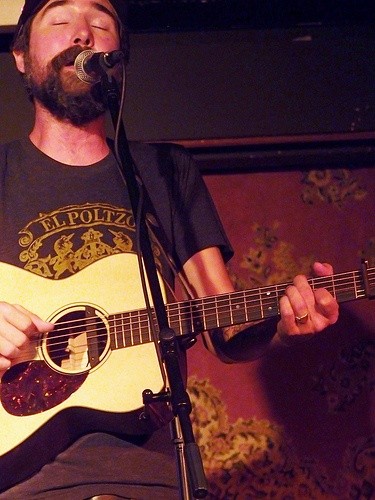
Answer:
[0,250,375,494]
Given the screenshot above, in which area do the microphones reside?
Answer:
[74,49,123,84]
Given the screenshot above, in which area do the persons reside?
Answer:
[0,0,341,500]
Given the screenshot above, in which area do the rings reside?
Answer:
[296,312,309,323]
